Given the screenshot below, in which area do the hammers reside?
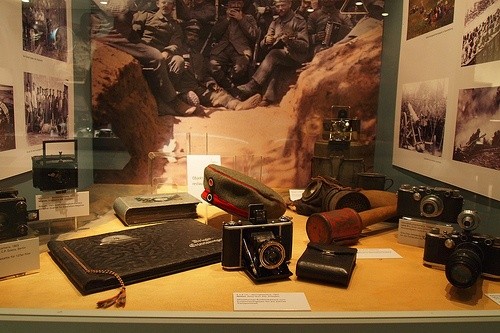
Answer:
[306,204,397,246]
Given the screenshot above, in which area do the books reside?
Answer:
[48,217,223,308]
[113,191,202,227]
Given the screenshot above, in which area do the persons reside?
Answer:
[470,128,480,148]
[25,82,68,133]
[419,100,431,139]
[90,0,354,116]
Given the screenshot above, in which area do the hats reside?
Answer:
[202,164,286,221]
[184,18,201,33]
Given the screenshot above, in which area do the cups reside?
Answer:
[358,172,393,192]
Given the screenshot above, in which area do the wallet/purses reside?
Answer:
[295,240,357,287]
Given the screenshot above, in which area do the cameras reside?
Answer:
[422,210,500,288]
[397,184,464,220]
[322,117,359,140]
[221,203,294,281]
